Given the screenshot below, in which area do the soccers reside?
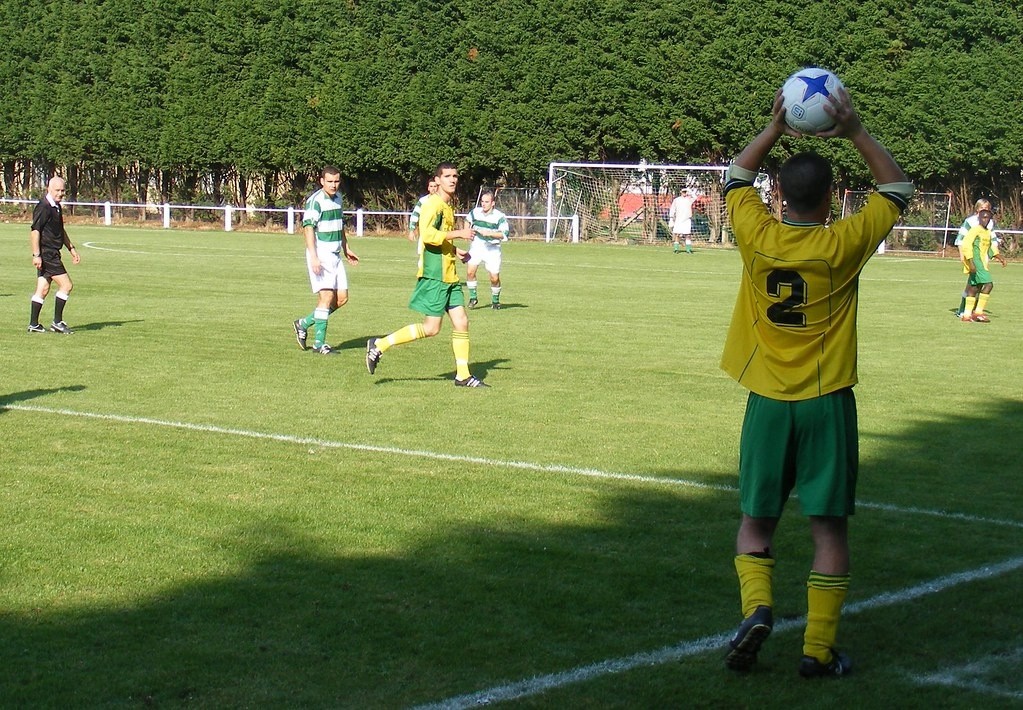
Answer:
[782,68,847,135]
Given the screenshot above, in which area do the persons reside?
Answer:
[291,166,359,355]
[719,86,916,679]
[408,179,438,268]
[464,193,510,310]
[669,188,697,254]
[27,176,80,335]
[366,162,490,388]
[955,198,1007,323]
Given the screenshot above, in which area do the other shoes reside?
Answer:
[686,251,693,254]
[491,302,501,310]
[467,298,478,309]
[957,311,990,323]
[674,250,678,254]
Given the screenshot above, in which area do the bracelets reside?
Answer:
[69,246,75,251]
[32,254,41,257]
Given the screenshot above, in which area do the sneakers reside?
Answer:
[28,324,49,333]
[313,343,340,354]
[798,649,850,678]
[292,319,308,351]
[455,374,492,388]
[367,338,381,374]
[50,321,75,335]
[727,606,773,670]
